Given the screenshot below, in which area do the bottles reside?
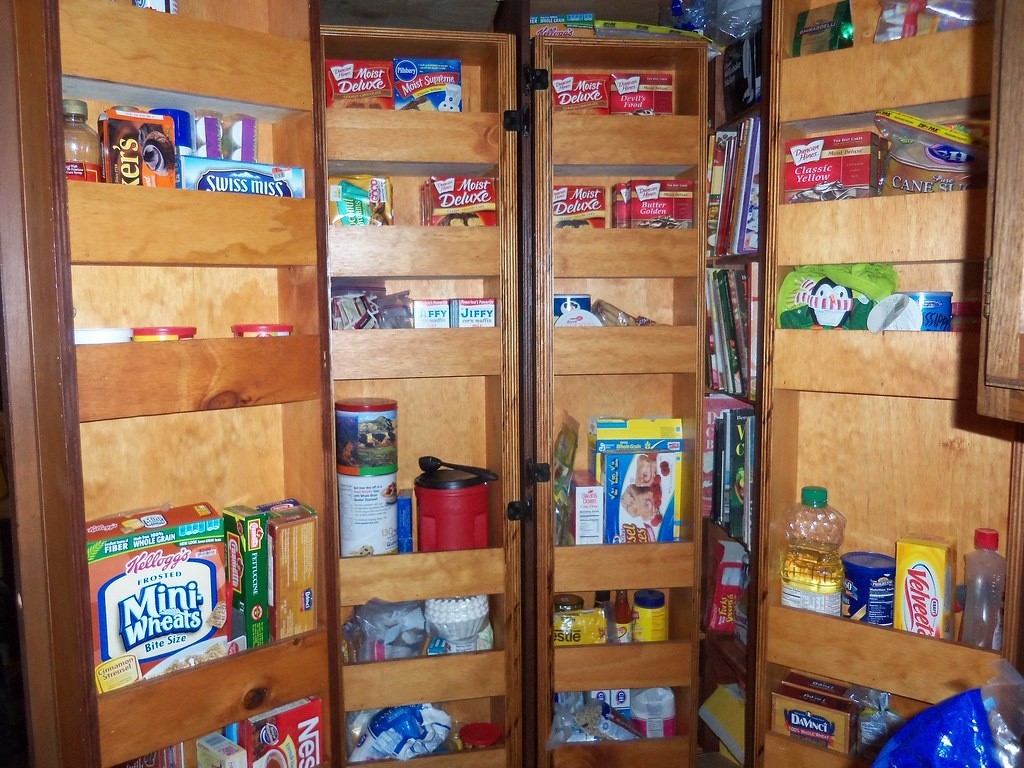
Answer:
[593,590,610,643]
[610,590,633,643]
[959,528,1006,650]
[62,98,103,182]
[780,485,847,616]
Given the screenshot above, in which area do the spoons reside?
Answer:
[419,456,499,481]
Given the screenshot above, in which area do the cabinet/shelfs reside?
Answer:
[755,0,1024,768]
[0,0,708,768]
[697,20,768,768]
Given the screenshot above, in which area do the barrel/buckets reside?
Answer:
[840,551,896,628]
[336,398,399,476]
[337,472,398,558]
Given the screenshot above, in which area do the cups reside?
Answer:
[446,721,465,752]
[415,469,489,553]
[632,589,668,641]
[553,594,584,613]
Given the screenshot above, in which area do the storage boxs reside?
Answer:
[85,502,237,695]
[611,688,630,741]
[703,540,751,633]
[553,185,607,228]
[612,183,628,228]
[589,690,611,741]
[429,175,498,228]
[781,673,865,711]
[722,38,758,122]
[892,533,958,639]
[412,300,450,329]
[266,505,317,641]
[448,298,496,328]
[771,686,859,755]
[224,723,239,746]
[239,694,324,768]
[708,55,728,130]
[568,470,603,546]
[98,109,177,187]
[627,179,695,228]
[196,732,247,768]
[784,132,890,204]
[420,184,425,226]
[394,57,463,112]
[600,448,677,544]
[325,58,396,110]
[588,416,684,541]
[255,497,316,514]
[397,488,415,553]
[531,11,594,40]
[175,155,305,198]
[553,73,613,115]
[423,181,431,226]
[223,506,271,650]
[611,73,673,115]
